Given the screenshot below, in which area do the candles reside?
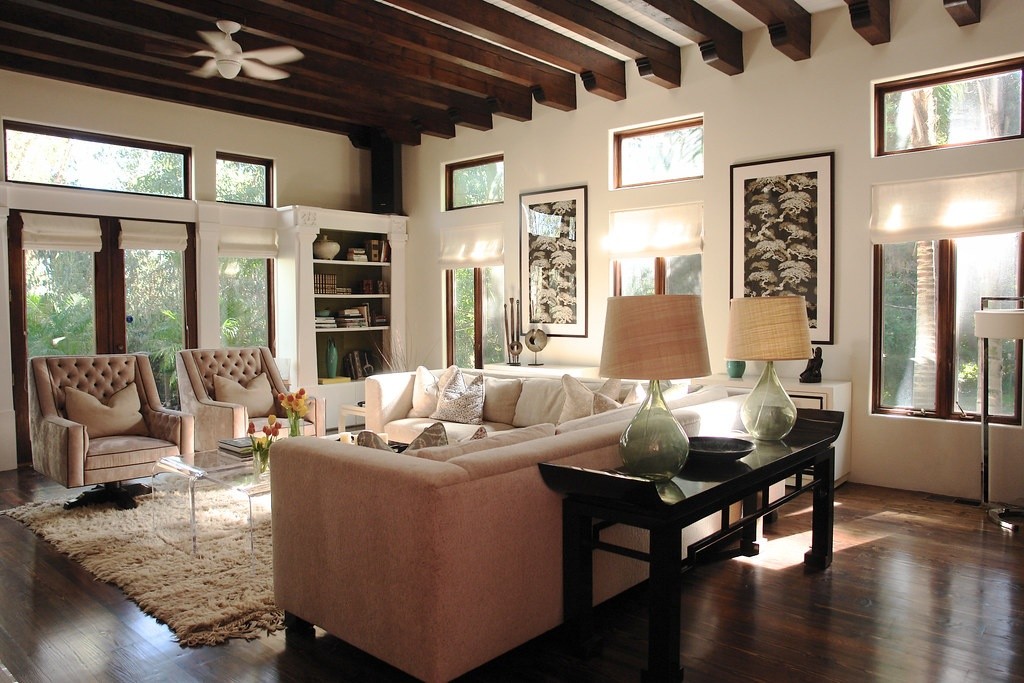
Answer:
[339,432,352,443]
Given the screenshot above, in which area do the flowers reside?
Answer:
[278,387,310,434]
[248,415,282,473]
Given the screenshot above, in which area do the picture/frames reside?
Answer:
[728,151,837,345]
[518,184,589,336]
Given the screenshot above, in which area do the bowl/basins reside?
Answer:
[673,437,755,463]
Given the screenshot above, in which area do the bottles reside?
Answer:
[313,235,341,260]
[327,342,337,378]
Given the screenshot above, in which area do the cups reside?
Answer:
[377,280,383,293]
[363,279,373,293]
[340,432,351,443]
[377,433,388,444]
[318,309,330,316]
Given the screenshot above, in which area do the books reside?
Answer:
[336,309,366,328]
[315,316,337,328]
[218,437,275,462]
[352,302,370,327]
[337,287,352,294]
[315,273,336,294]
[356,279,389,293]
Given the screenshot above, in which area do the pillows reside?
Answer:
[211,374,276,419]
[63,382,151,438]
[358,362,652,461]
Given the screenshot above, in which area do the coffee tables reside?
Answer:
[152,437,335,560]
[339,405,366,437]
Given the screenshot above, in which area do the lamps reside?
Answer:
[214,52,242,80]
[724,297,813,441]
[597,294,711,487]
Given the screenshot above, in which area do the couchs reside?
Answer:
[26,352,194,509]
[175,346,326,455]
[364,368,686,444]
[271,390,755,681]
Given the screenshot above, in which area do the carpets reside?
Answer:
[0,471,287,647]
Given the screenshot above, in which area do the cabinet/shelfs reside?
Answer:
[722,377,852,491]
[278,204,408,430]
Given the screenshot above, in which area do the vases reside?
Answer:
[328,340,338,379]
[252,449,270,477]
[289,419,305,438]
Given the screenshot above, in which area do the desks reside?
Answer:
[538,407,846,682]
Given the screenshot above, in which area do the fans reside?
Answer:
[180,21,303,81]
